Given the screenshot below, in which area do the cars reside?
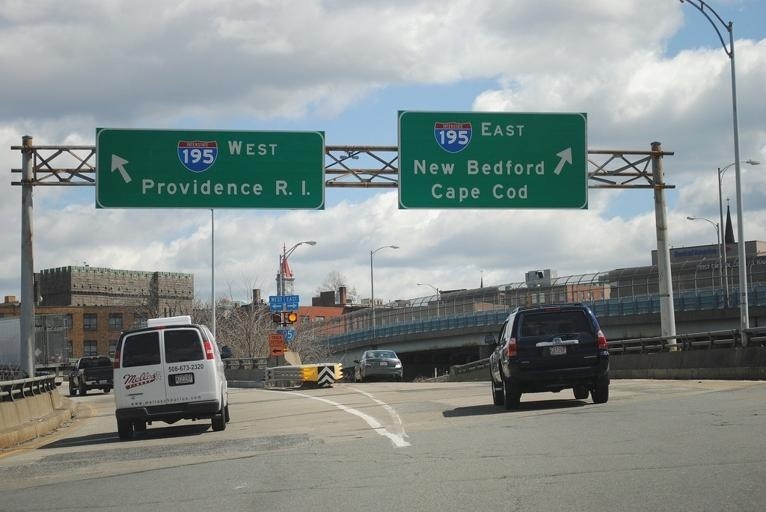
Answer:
[353,348,404,383]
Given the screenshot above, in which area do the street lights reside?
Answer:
[370,245,400,345]
[686,215,725,306]
[717,159,761,308]
[279,240,316,352]
[270,312,282,324]
[416,282,441,319]
[287,311,297,321]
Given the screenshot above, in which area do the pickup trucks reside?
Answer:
[67,355,114,396]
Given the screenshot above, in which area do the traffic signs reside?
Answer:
[95,127,325,210]
[268,334,284,356]
[397,110,588,211]
[267,296,283,311]
[285,295,299,309]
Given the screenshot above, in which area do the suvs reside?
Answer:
[484,301,611,412]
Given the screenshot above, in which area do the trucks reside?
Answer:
[0,314,69,387]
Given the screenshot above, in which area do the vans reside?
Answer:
[112,316,232,440]
